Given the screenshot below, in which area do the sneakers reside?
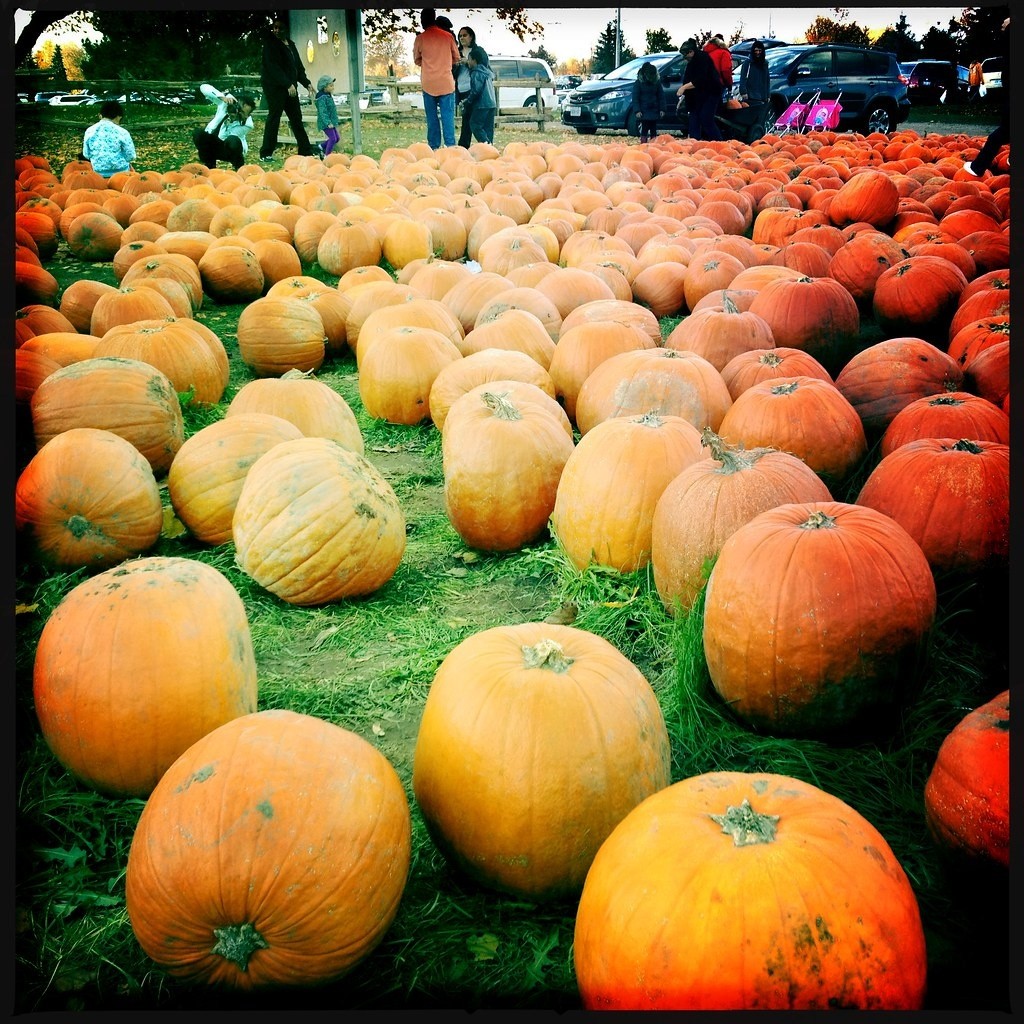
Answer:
[258,155,277,163]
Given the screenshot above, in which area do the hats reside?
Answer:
[317,75,336,91]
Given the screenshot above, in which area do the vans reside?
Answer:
[383,56,558,113]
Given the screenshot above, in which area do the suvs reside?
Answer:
[981,57,1004,98]
[907,59,970,108]
[676,42,912,137]
[556,75,584,90]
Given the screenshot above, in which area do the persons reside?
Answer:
[788,108,800,122]
[739,41,770,138]
[633,62,665,145]
[815,108,828,124]
[413,5,499,154]
[259,18,317,163]
[314,75,340,162]
[963,120,1010,177]
[193,84,256,171]
[677,38,723,142]
[703,34,733,107]
[82,101,136,179]
[967,52,985,111]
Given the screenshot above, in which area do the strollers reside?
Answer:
[765,87,844,135]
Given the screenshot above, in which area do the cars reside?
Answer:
[561,51,751,137]
[299,90,384,105]
[897,61,917,77]
[19,90,195,106]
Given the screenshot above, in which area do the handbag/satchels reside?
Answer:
[938,89,947,105]
[978,84,987,98]
[358,94,369,109]
[304,87,318,115]
[967,84,971,93]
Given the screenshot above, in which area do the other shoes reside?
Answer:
[963,161,978,176]
[317,144,329,160]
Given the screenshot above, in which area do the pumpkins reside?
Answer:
[18,130,1010,1013]
[727,100,749,109]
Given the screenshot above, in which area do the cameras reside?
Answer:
[227,100,242,114]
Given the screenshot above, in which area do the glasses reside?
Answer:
[459,34,468,37]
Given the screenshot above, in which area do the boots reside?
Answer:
[641,136,647,144]
[650,136,656,140]
